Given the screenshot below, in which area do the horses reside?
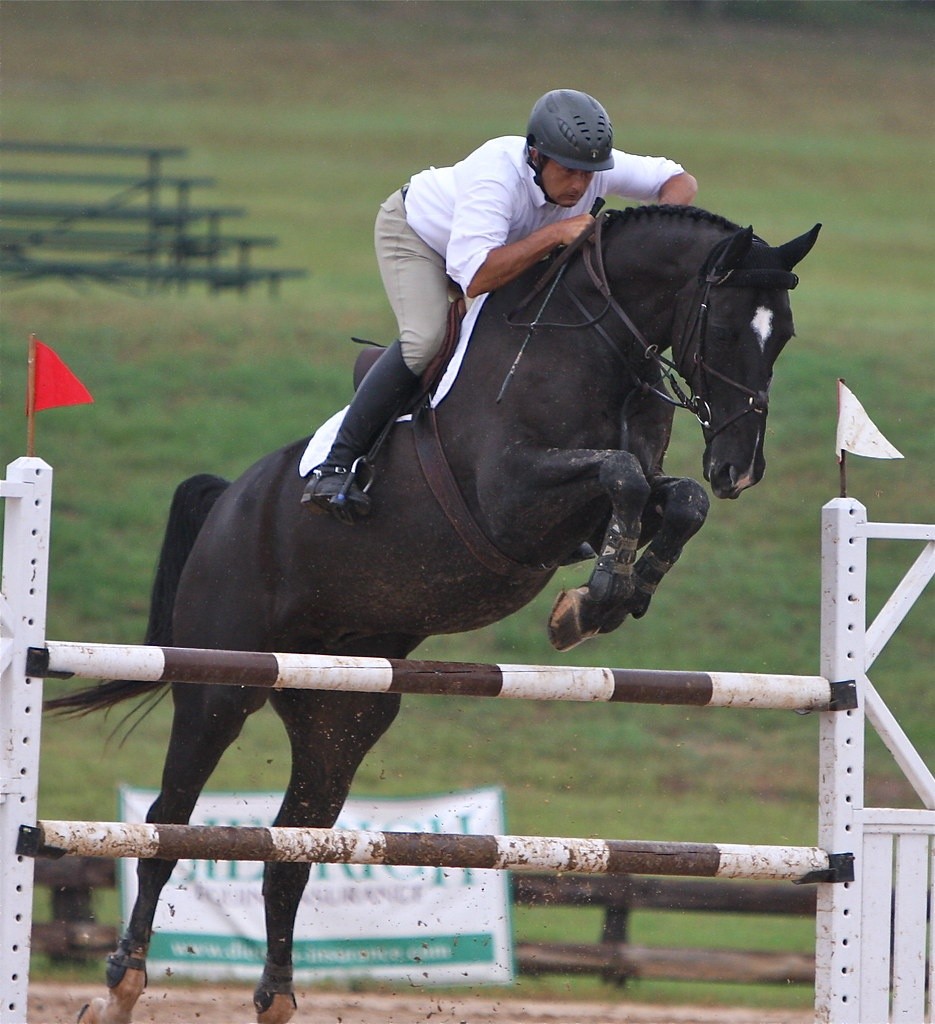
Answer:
[42,203,823,1024]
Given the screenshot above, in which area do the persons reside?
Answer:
[298,90,699,567]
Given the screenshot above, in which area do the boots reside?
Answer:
[300,338,420,526]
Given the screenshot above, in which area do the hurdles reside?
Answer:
[1,456,934,1024]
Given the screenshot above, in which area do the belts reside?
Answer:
[399,183,407,198]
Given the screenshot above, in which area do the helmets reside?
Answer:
[526,89,615,171]
